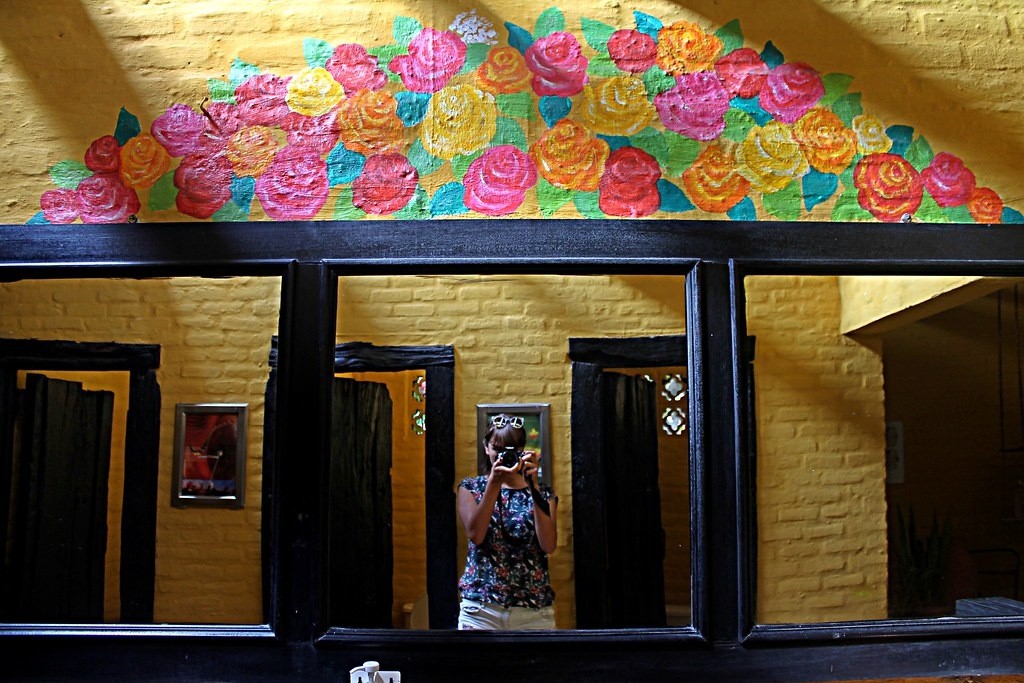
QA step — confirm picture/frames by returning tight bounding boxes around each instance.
[171,403,250,509]
[478,403,554,492]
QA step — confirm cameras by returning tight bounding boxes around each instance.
[498,446,531,475]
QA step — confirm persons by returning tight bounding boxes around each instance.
[457,413,559,629]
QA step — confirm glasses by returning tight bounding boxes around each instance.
[492,414,526,430]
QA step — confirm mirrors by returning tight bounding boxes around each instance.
[1,256,296,644]
[727,259,1024,647]
[309,256,706,650]
